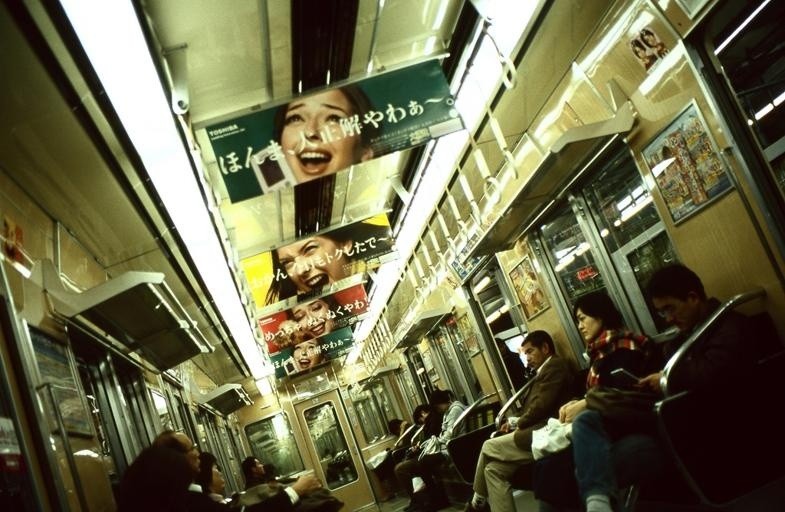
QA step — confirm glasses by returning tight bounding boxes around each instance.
[184,443,199,454]
[657,298,684,319]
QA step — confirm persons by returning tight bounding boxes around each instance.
[269,227,379,294]
[110,427,345,512]
[640,28,669,58]
[631,39,657,70]
[277,86,373,180]
[290,335,325,374]
[384,257,785,510]
[277,296,347,346]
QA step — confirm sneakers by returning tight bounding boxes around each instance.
[411,476,426,493]
[404,496,449,512]
[380,491,396,502]
